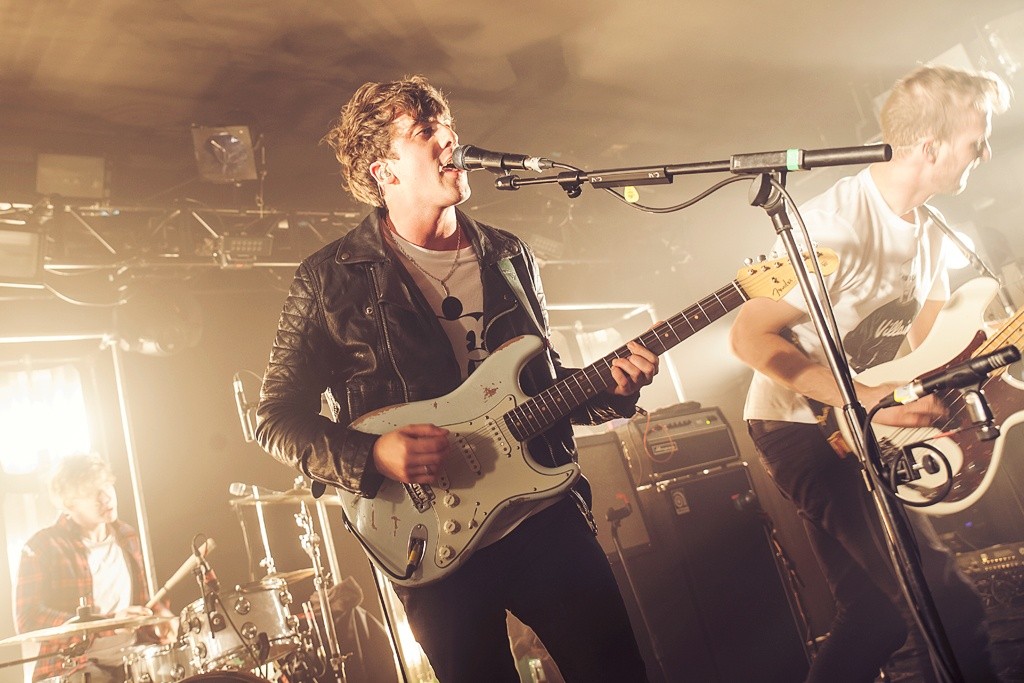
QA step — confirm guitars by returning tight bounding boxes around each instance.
[333,239,843,588]
[870,301,1024,519]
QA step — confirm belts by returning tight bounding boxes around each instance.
[750,420,793,440]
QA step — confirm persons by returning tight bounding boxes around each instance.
[254,74,659,683]
[15,451,179,683]
[729,66,1013,683]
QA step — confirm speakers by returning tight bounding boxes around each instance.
[569,431,816,682]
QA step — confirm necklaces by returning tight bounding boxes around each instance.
[388,222,463,320]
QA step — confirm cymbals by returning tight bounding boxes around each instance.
[0,612,174,650]
[261,563,327,585]
[227,488,341,508]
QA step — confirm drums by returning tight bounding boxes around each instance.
[179,576,300,672]
[120,642,201,683]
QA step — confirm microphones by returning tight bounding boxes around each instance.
[229,483,284,498]
[190,545,220,591]
[604,504,633,520]
[879,344,1022,408]
[451,144,555,173]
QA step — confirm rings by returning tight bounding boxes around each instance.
[426,465,431,474]
